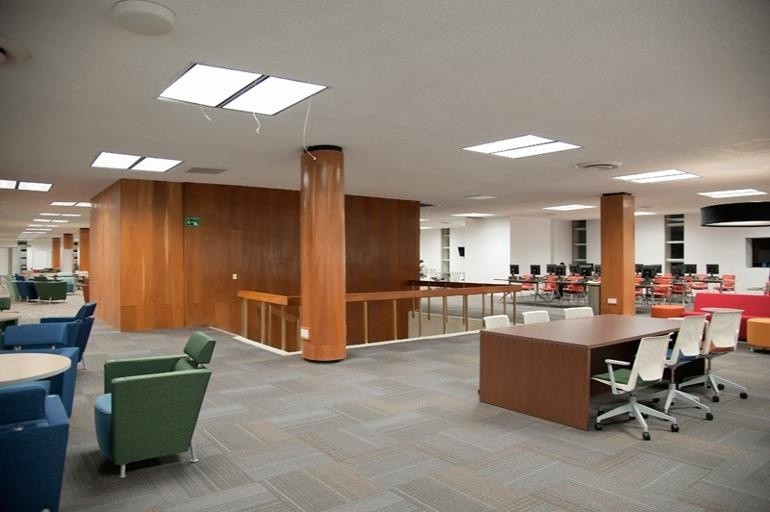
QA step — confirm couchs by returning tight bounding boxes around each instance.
[684,291,770,340]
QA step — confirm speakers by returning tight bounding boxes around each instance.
[458,247,464,257]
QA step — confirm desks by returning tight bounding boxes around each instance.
[0,311,20,326]
[0,353,73,386]
[479,314,712,433]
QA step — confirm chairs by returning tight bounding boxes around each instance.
[493,270,736,310]
[0,297,10,312]
[0,322,80,419]
[483,306,593,330]
[40,302,97,370]
[0,379,70,511]
[93,331,217,479]
[5,272,67,305]
[590,309,750,441]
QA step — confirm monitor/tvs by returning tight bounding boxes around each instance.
[635,264,719,285]
[508,264,601,282]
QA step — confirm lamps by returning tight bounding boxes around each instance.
[700,201,770,227]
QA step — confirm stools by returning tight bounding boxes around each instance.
[651,303,686,318]
[747,317,770,352]
